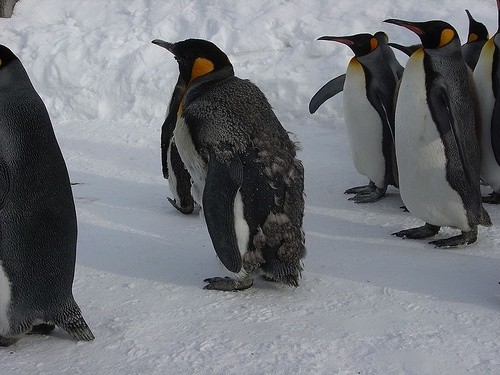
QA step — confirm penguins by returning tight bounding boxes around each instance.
[309,0,500,251]
[147,37,308,292]
[0,45,96,348]
